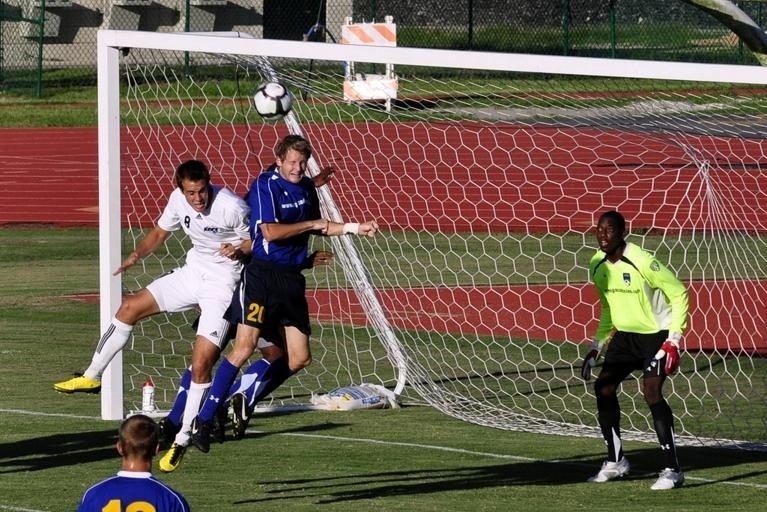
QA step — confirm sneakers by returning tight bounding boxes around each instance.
[52,373,102,395]
[158,393,254,474]
[650,468,684,490]
[587,456,630,483]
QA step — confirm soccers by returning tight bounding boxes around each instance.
[252,81,292,121]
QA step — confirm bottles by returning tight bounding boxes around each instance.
[141,379,156,413]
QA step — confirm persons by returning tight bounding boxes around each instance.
[78,415,191,512]
[582,209,689,493]
[51,161,250,472]
[163,163,341,443]
[192,136,379,453]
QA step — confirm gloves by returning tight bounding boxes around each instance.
[655,332,681,375]
[581,339,604,381]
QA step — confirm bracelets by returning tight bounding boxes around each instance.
[344,223,360,236]
